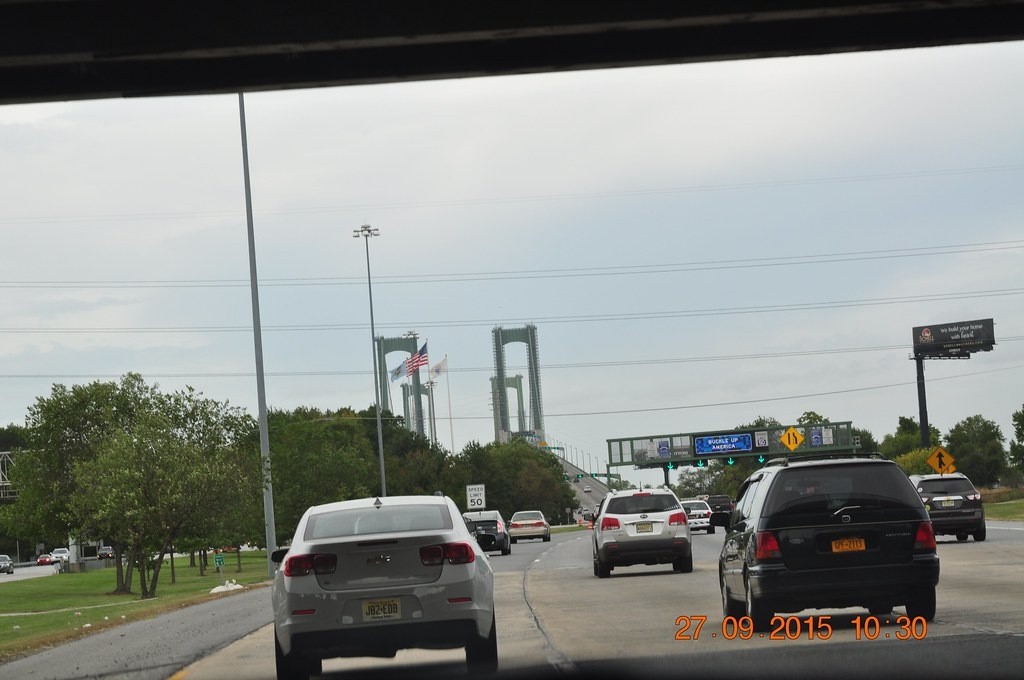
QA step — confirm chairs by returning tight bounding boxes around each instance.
[772,491,801,516]
[654,497,667,509]
[321,516,356,537]
[404,514,443,532]
[612,501,628,515]
[943,483,959,493]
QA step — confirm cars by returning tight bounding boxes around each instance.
[461,510,512,555]
[269,490,499,680]
[37,554,53,566]
[507,510,551,543]
[680,500,715,534]
[96,546,115,560]
[0,555,14,574]
[50,548,70,564]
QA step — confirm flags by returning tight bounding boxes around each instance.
[406,343,428,376]
[389,360,407,382]
[430,357,446,379]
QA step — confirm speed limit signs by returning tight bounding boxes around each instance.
[467,484,487,509]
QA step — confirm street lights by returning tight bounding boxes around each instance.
[352,224,387,497]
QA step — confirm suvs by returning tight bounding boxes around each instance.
[908,472,987,542]
[583,486,694,578]
[706,494,736,527]
[708,448,943,630]
